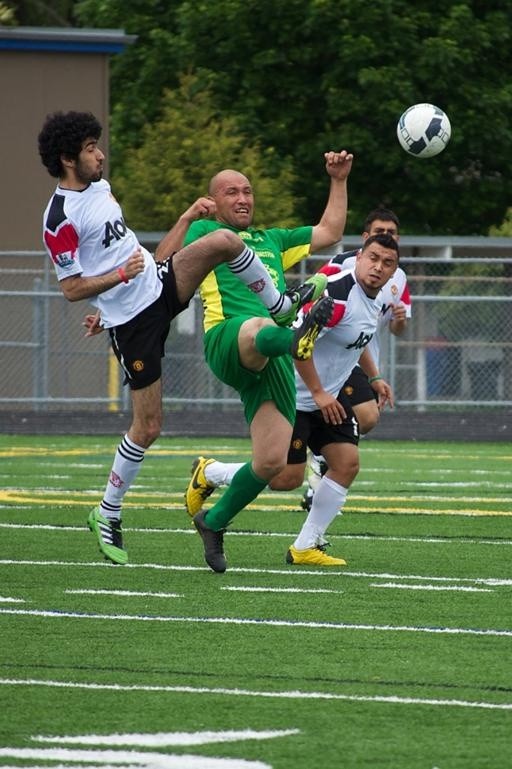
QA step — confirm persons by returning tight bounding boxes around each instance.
[300,204,414,516]
[184,237,400,567]
[154,147,355,573]
[37,110,328,567]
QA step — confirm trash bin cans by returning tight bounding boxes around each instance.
[426,335,512,400]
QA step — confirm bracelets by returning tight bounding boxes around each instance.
[117,266,129,285]
[368,375,383,385]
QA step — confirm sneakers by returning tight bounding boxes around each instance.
[192,508,228,573]
[273,272,328,327]
[184,455,218,518]
[86,505,129,567]
[286,545,346,567]
[301,454,346,518]
[290,293,334,362]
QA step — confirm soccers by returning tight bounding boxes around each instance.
[397,103,451,158]
